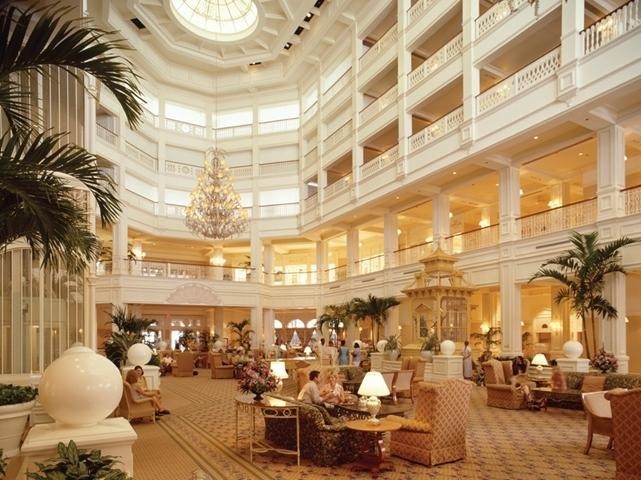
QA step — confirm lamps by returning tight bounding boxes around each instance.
[269,362,289,392]
[183,45,251,240]
[303,346,312,356]
[356,372,391,423]
[532,354,549,376]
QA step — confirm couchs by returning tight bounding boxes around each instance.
[208,350,235,379]
[481,359,536,410]
[387,378,473,467]
[297,366,345,398]
[551,372,641,407]
[262,395,376,466]
[399,356,425,397]
[324,365,367,390]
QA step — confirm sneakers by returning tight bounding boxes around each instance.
[159,409,169,414]
[155,411,160,420]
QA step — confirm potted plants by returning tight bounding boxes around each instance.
[0,382,38,459]
[417,334,438,362]
[382,334,402,361]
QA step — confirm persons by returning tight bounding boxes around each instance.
[126,369,170,417]
[325,341,339,367]
[208,338,255,380]
[351,343,361,368]
[461,341,472,378]
[319,371,344,407]
[549,359,566,389]
[297,371,334,418]
[162,353,173,370]
[317,339,325,356]
[134,366,164,420]
[273,339,292,359]
[513,355,526,375]
[338,340,350,366]
[510,367,537,404]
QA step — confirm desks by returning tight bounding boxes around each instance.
[344,377,364,393]
[345,419,400,479]
[232,393,303,468]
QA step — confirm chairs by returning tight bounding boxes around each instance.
[605,388,640,480]
[509,358,529,386]
[581,390,627,456]
[115,381,156,426]
[379,370,417,405]
[138,376,163,411]
[172,352,193,377]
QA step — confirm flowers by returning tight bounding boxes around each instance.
[588,348,618,371]
[238,359,280,393]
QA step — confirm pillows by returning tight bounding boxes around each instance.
[222,359,231,365]
[307,402,334,425]
[581,376,607,392]
[347,369,361,380]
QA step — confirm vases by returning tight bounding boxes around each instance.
[253,388,265,400]
